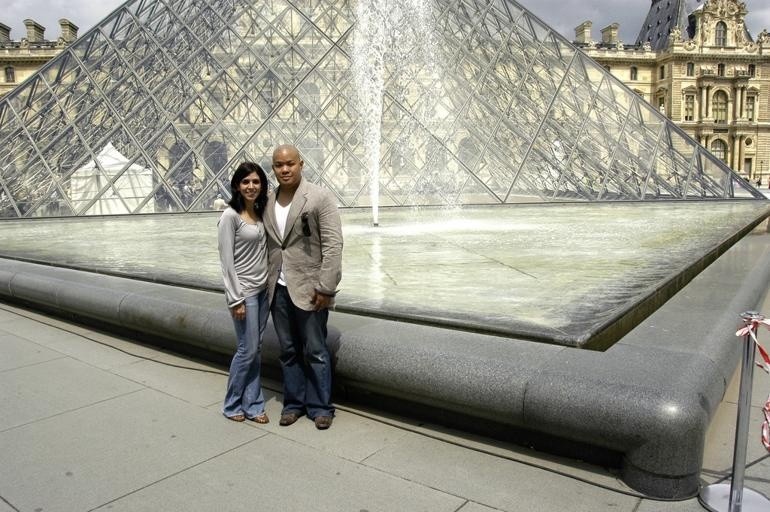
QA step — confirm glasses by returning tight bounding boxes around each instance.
[301,212,312,237]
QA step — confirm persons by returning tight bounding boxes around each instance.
[153,179,233,212]
[218,161,271,425]
[258,144,344,430]
[50,189,59,211]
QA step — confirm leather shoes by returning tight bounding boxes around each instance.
[280,413,298,425]
[252,413,269,423]
[315,415,333,429]
[230,416,245,422]
